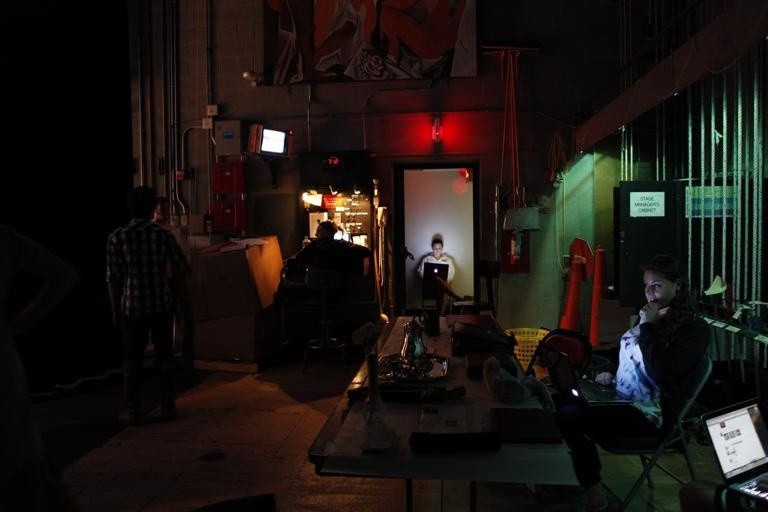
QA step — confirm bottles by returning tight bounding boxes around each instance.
[302,236,311,247]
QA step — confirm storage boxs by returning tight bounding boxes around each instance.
[185,157,372,363]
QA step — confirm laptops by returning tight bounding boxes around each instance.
[560,352,634,407]
[423,263,449,281]
[700,396,768,504]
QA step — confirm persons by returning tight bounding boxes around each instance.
[287,221,371,275]
[544,255,711,512]
[107,186,189,424]
[417,239,470,317]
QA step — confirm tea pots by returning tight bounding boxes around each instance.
[401,315,427,358]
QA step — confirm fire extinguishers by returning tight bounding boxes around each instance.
[510,230,525,265]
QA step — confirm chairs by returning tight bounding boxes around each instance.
[503,328,552,383]
[586,355,714,512]
[422,295,453,315]
[525,328,593,379]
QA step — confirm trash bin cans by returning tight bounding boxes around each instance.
[505,328,551,376]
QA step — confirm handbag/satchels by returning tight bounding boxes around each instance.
[503,207,541,230]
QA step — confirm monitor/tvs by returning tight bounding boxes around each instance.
[624,185,672,226]
[247,124,297,158]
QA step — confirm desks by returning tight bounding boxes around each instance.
[309,313,581,512]
